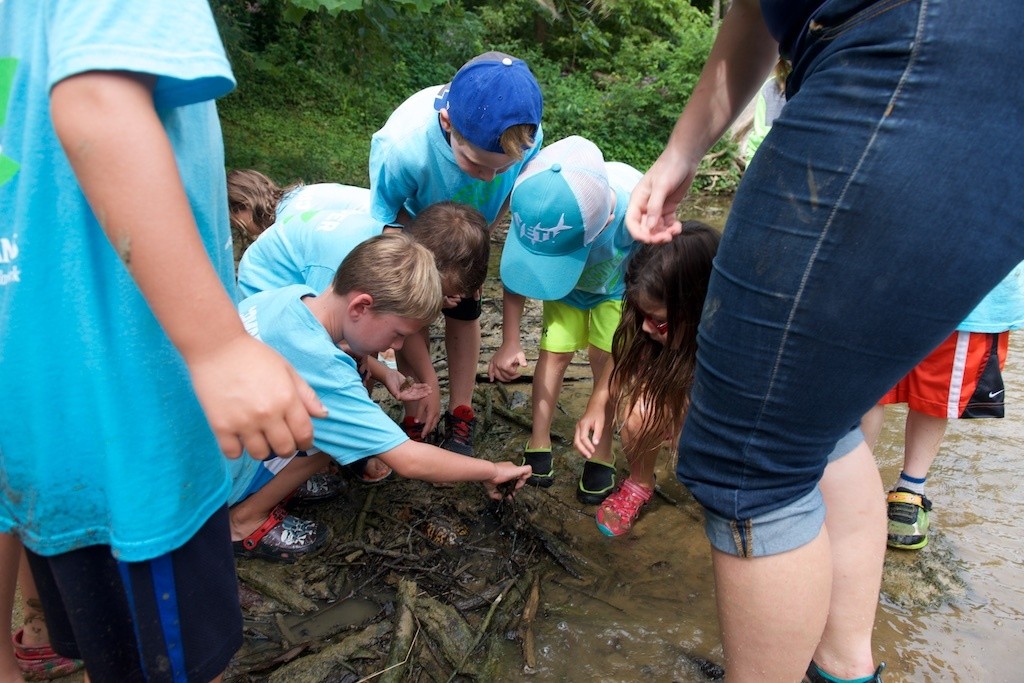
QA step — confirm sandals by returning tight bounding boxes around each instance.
[595,479,653,537]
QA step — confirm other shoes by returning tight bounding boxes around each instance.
[13,628,82,680]
[577,449,618,505]
[521,439,555,488]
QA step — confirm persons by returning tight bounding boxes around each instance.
[626,1,1024,683]
[227,168,370,249]
[237,199,491,483]
[0,0,329,683]
[369,51,544,485]
[860,258,1024,550]
[488,136,647,506]
[227,235,531,562]
[0,531,85,683]
[574,220,722,537]
[731,57,789,162]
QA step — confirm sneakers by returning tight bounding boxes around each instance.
[887,487,934,550]
[439,405,478,459]
[399,416,428,443]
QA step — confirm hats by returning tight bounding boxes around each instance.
[500,134,610,301]
[434,50,544,154]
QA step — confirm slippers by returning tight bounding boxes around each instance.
[287,475,340,502]
[351,456,393,483]
[232,504,327,561]
